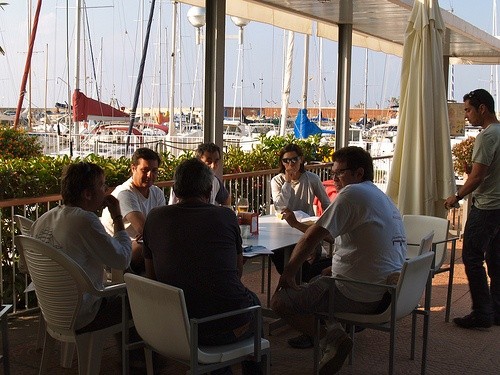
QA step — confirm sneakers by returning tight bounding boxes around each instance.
[288,334,315,348]
[317,327,353,375]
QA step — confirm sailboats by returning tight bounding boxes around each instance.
[0,1,499,167]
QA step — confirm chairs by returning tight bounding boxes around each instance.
[122,270,271,375]
[13,235,154,374]
[396,213,456,325]
[303,252,434,374]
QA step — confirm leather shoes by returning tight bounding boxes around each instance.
[452,312,493,329]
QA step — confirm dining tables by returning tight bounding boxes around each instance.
[124,212,342,349]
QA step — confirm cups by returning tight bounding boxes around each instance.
[240,225,252,248]
[237,198,249,213]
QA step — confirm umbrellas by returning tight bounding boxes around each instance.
[385,1,457,223]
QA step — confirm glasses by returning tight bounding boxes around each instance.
[468,91,481,106]
[330,168,353,177]
[281,158,313,163]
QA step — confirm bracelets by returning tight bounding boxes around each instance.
[455,192,461,202]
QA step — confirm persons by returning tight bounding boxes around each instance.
[168,143,232,214]
[270,146,408,375]
[443,89,500,328]
[98,147,162,360]
[268,145,332,310]
[31,162,165,375]
[143,158,267,375]
